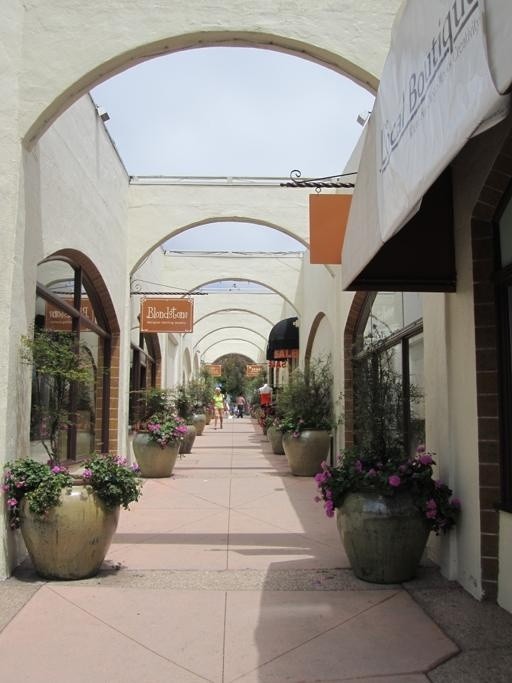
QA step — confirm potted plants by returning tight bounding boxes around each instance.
[269,363,331,478]
[177,379,213,454]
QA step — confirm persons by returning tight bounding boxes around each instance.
[223,394,233,419]
[235,392,247,418]
[210,386,228,431]
[258,383,273,405]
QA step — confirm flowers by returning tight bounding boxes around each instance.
[316,445,458,539]
[2,450,140,532]
[144,414,190,450]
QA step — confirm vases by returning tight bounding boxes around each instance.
[17,480,121,585]
[336,489,433,587]
[133,431,182,480]
